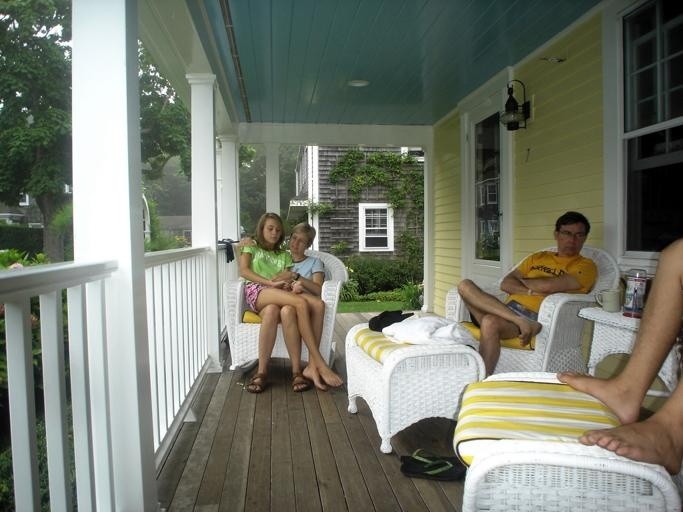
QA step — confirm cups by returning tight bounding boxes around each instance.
[596,290,620,313]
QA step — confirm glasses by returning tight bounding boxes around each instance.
[290,236,309,246]
[557,230,586,240]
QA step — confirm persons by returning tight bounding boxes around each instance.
[244,222,325,394]
[459,212,596,375]
[238,212,342,391]
[557,237,682,475]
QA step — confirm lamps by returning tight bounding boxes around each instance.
[500,79,532,131]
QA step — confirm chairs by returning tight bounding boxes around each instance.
[445,245,620,377]
[224,248,348,373]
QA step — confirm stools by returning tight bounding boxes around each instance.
[342,323,486,454]
[450,370,680,512]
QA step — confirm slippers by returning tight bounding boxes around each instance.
[400,459,466,481]
[401,449,461,465]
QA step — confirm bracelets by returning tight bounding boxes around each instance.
[525,288,533,294]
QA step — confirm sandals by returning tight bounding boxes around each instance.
[292,373,312,392]
[247,373,267,393]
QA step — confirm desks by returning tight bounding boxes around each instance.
[579,306,682,394]
